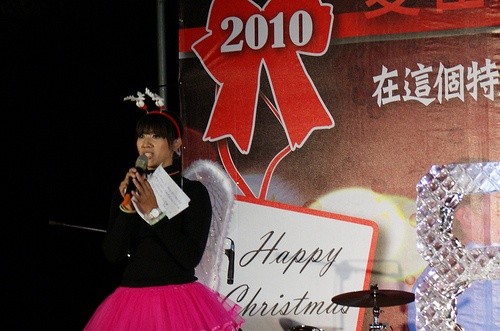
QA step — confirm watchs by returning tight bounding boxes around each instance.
[146,207,162,221]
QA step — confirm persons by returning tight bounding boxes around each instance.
[85,88,245,331]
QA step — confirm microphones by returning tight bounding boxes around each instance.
[123,155,148,205]
